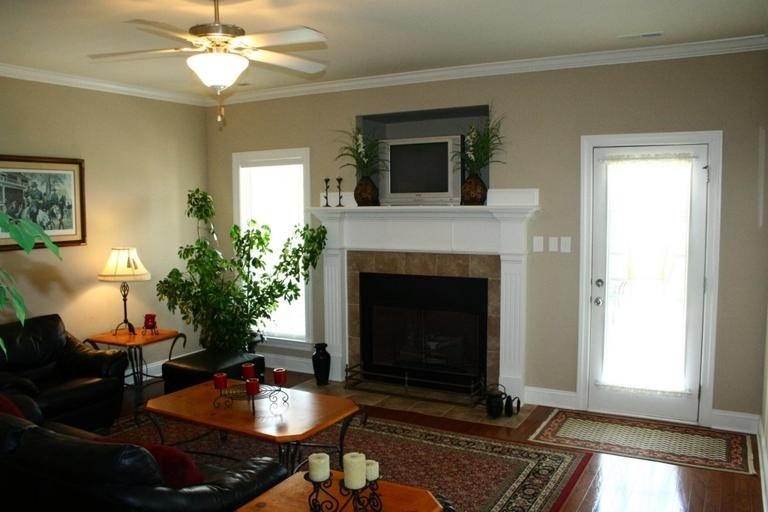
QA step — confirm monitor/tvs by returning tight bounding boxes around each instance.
[377,136,461,207]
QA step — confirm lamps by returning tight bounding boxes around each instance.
[187,52,250,132]
[96,247,151,336]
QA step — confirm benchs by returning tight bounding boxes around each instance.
[162,349,265,394]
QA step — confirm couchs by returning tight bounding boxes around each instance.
[1,393,288,511]
[0,313,129,429]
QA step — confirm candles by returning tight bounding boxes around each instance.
[273,368,285,384]
[213,373,227,389]
[343,452,366,490]
[246,378,259,395]
[145,315,155,328]
[242,363,255,376]
[366,460,378,481]
[308,454,330,482]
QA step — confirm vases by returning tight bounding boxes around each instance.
[461,174,487,206]
[312,343,330,385]
[353,176,378,206]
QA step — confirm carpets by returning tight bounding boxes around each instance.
[528,407,757,475]
[90,404,592,512]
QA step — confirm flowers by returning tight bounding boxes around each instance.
[450,98,508,173]
[334,126,393,178]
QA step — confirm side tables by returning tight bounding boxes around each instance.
[235,470,443,511]
[83,327,187,404]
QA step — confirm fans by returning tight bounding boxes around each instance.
[85,1,329,76]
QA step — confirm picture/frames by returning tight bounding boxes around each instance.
[1,154,86,252]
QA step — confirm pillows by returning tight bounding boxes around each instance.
[39,420,205,488]
[0,394,24,417]
